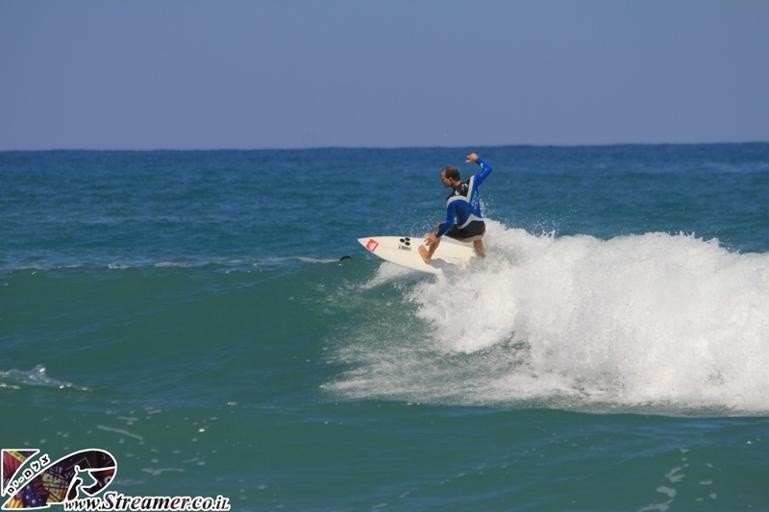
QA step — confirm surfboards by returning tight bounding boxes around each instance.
[358,236,475,275]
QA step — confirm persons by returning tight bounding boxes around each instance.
[418,152,492,264]
